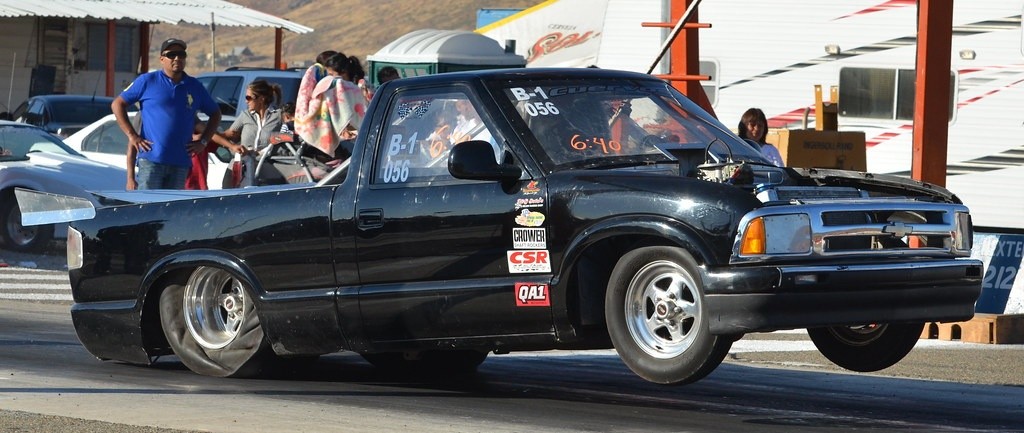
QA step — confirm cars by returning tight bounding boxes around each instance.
[0,61,309,255]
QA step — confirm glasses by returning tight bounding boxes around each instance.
[161,51,186,59]
[246,95,259,100]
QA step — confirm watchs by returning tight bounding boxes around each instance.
[200,139,207,145]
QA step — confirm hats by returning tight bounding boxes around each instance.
[160,38,186,53]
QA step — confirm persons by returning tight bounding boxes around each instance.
[111,38,223,189]
[738,108,784,166]
[127,50,502,191]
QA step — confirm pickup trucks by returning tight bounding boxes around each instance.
[65,65,985,387]
